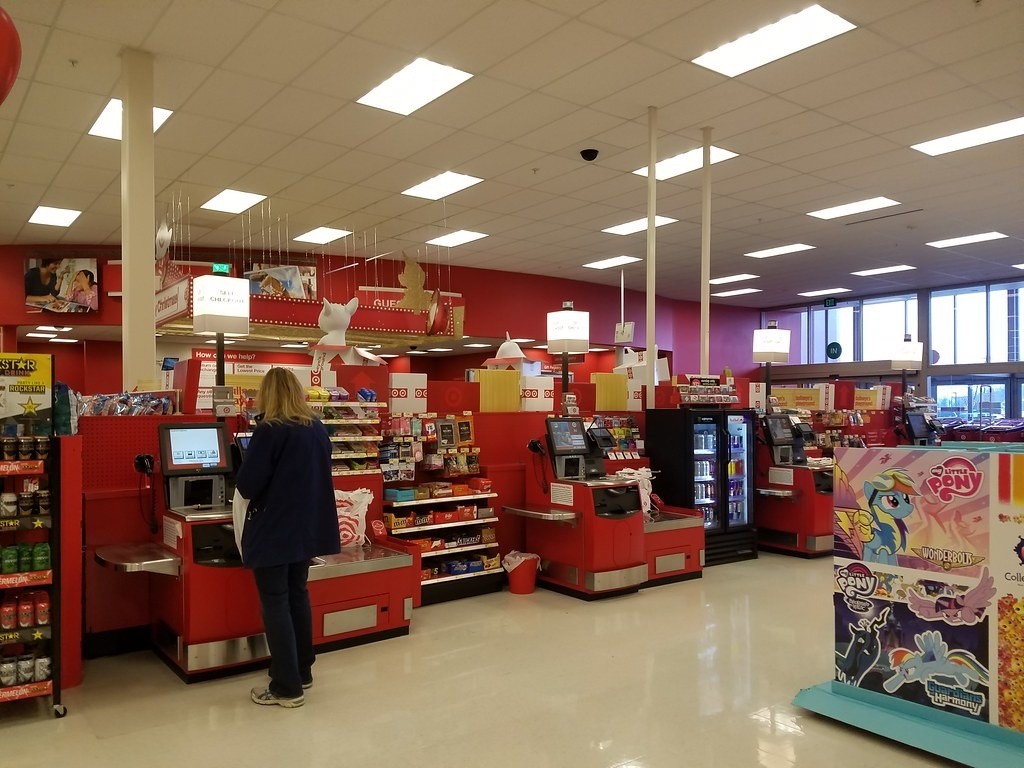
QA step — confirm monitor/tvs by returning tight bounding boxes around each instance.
[764,415,796,446]
[545,418,590,455]
[159,423,233,476]
[907,412,930,438]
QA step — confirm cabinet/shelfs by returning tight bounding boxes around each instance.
[0,355,1024,768]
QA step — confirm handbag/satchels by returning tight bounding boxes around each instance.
[334,487,374,548]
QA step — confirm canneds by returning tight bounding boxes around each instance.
[694,432,741,523]
[0,437,52,688]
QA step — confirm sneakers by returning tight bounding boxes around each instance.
[252,687,305,708]
[302,680,313,689]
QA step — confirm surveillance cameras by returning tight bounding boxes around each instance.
[580,148,599,161]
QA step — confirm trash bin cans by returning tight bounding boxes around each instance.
[505,551,539,595]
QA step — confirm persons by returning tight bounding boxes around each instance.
[229,366,342,709]
[67,269,98,311]
[24,257,70,303]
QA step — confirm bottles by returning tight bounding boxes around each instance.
[830,408,868,426]
[826,430,868,448]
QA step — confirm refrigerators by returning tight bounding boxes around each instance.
[644,407,760,567]
